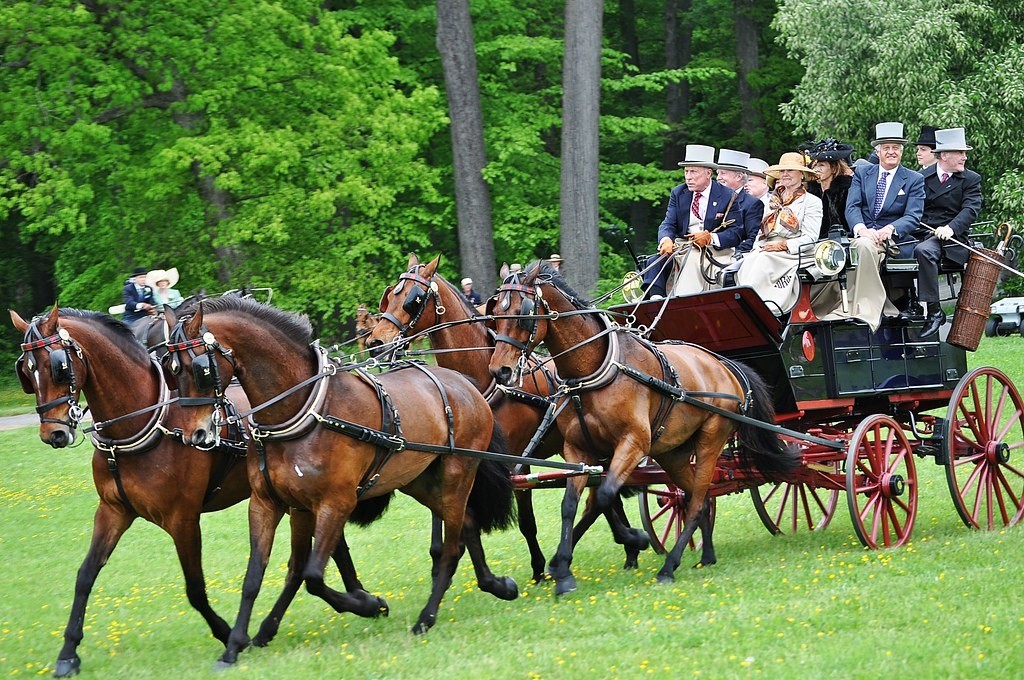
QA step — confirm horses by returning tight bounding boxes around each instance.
[96,390,111,400]
[8,301,390,675]
[489,260,803,596]
[367,251,652,595]
[149,295,520,667]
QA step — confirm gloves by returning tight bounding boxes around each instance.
[764,239,787,252]
[688,230,711,248]
[934,225,954,240]
[659,239,675,257]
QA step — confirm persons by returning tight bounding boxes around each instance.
[145,268,183,311]
[461,278,482,306]
[122,267,158,348]
[547,254,564,269]
[644,122,982,339]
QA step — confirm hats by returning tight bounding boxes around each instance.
[545,254,564,262]
[763,151,817,179]
[461,278,472,286]
[912,126,942,144]
[749,158,769,179]
[931,128,973,152]
[145,267,179,288]
[717,149,752,173]
[678,145,718,169]
[870,122,908,148]
[130,267,148,277]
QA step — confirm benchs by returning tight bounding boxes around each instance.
[636,221,991,324]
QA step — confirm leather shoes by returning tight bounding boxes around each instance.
[918,309,946,340]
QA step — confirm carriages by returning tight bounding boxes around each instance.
[8,232,1023,680]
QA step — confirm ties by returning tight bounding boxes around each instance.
[691,192,704,221]
[873,172,890,219]
[940,173,949,184]
